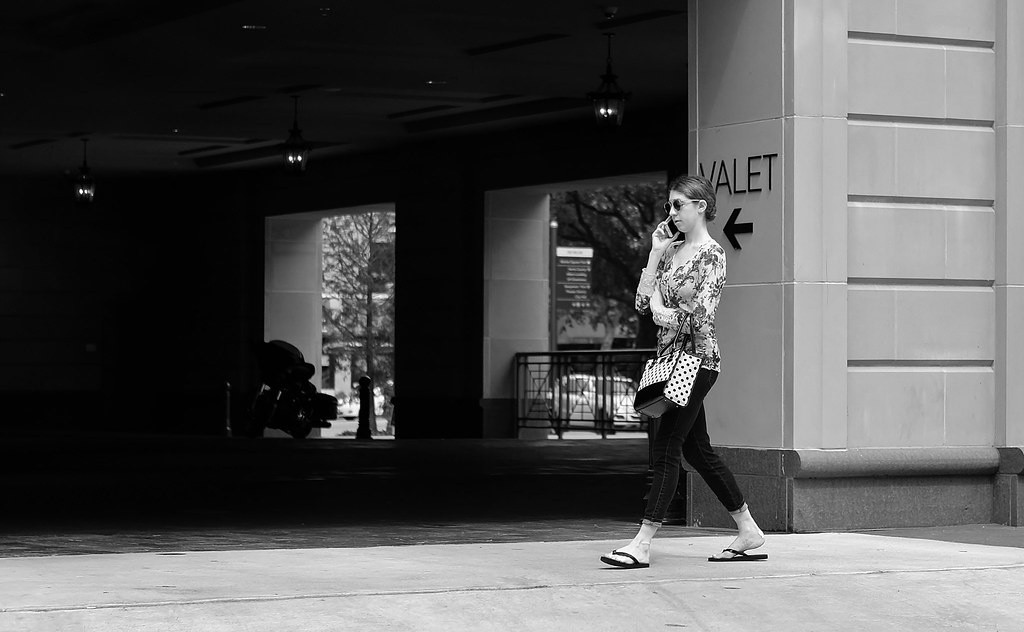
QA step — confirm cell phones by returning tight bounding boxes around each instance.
[665,218,678,238]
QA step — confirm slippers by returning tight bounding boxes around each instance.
[708,548,768,561]
[601,550,649,568]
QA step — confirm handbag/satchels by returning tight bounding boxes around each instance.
[634,313,703,418]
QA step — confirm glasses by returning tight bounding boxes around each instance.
[664,198,700,214]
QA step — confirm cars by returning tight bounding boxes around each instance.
[329,390,385,420]
[545,373,650,433]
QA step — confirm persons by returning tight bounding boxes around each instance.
[601,173,770,568]
